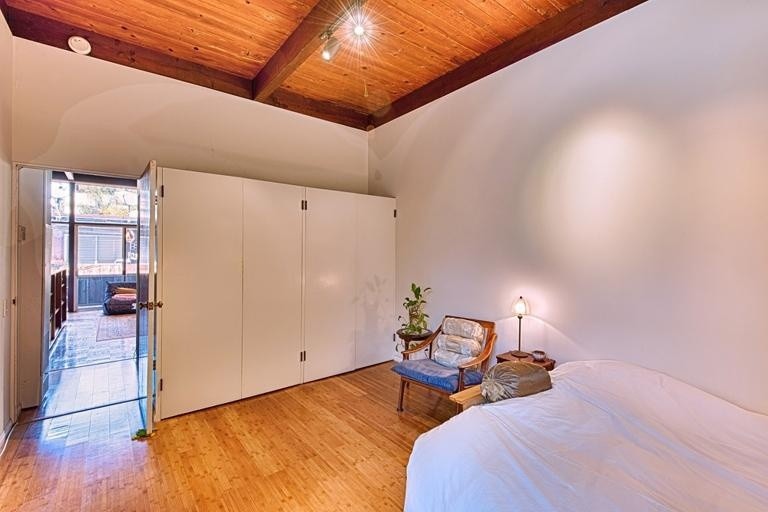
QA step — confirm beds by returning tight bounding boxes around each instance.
[400,355,767,512]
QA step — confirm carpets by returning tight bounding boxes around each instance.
[96,313,148,343]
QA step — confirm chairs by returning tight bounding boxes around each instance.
[102,281,137,315]
[390,315,495,417]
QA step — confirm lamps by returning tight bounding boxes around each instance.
[508,294,530,358]
[317,29,340,61]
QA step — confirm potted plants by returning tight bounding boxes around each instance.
[394,283,428,339]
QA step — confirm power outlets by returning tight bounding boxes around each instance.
[424,345,429,353]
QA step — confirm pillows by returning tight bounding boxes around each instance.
[111,294,136,301]
[432,346,479,370]
[438,333,480,356]
[112,286,137,294]
[441,317,485,343]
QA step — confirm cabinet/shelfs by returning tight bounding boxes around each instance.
[157,166,304,425]
[49,267,68,346]
[303,184,396,383]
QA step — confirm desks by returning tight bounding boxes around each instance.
[395,328,431,388]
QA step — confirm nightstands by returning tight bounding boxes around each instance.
[495,352,554,371]
